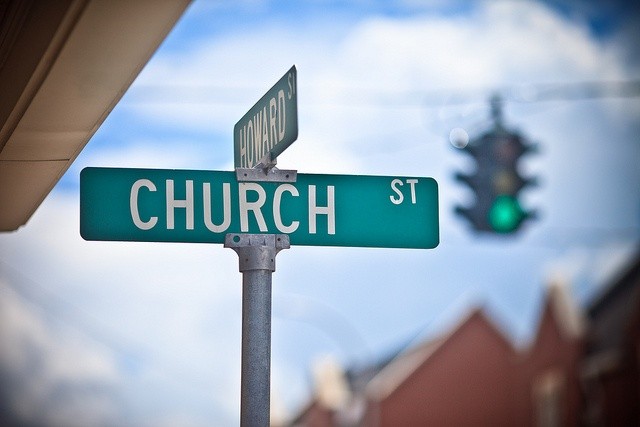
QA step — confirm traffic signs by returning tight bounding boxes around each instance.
[233,64,298,169]
[79,165,438,248]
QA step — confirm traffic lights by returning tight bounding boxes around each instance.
[455,127,539,233]
